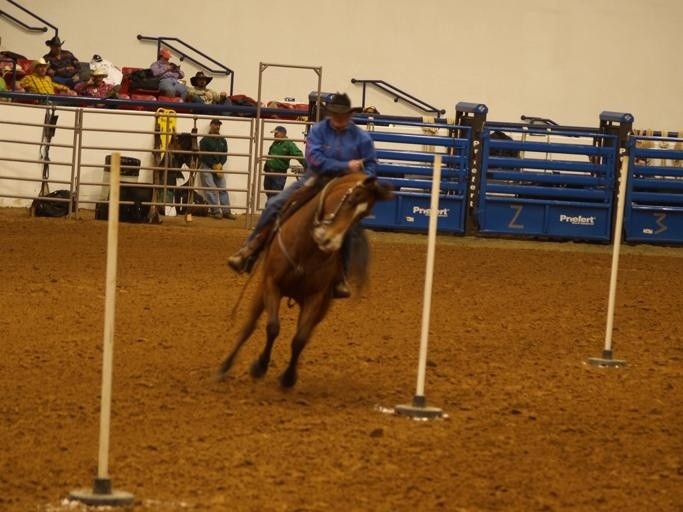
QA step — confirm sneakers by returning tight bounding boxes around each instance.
[229,251,246,270]
[334,282,352,297]
[215,213,236,219]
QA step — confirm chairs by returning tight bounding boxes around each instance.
[0,55,309,120]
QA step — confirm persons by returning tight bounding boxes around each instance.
[0,36,233,115]
[229,92,378,298]
[200,119,236,220]
[263,126,308,201]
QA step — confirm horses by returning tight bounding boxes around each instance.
[212,169,397,393]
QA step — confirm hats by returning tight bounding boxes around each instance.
[271,126,286,133]
[321,94,363,114]
[211,119,222,125]
[91,68,108,77]
[32,58,50,69]
[46,37,64,45]
[191,72,212,85]
[160,48,171,58]
